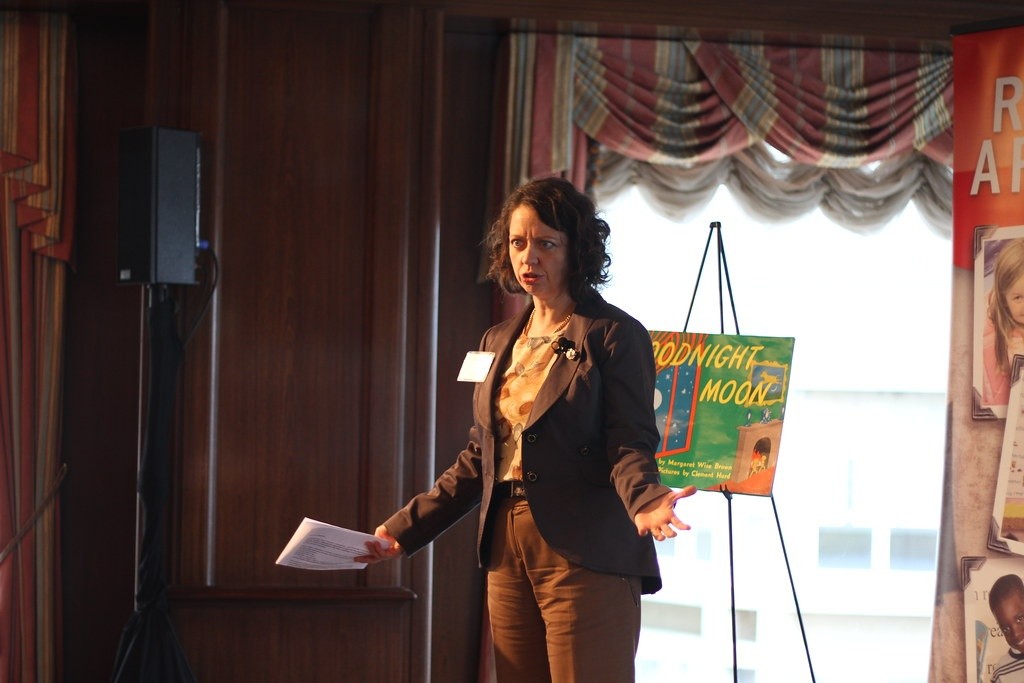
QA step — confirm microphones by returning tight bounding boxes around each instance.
[554,337,568,354]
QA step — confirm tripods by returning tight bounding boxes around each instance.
[111,282,195,682]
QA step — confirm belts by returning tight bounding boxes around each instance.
[496,481,525,498]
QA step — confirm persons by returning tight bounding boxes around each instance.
[347,175,701,682]
[985,572,1024,683]
[982,235,1024,407]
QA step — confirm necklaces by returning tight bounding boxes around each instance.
[523,304,575,337]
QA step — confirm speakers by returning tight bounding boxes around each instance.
[118,124,202,285]
